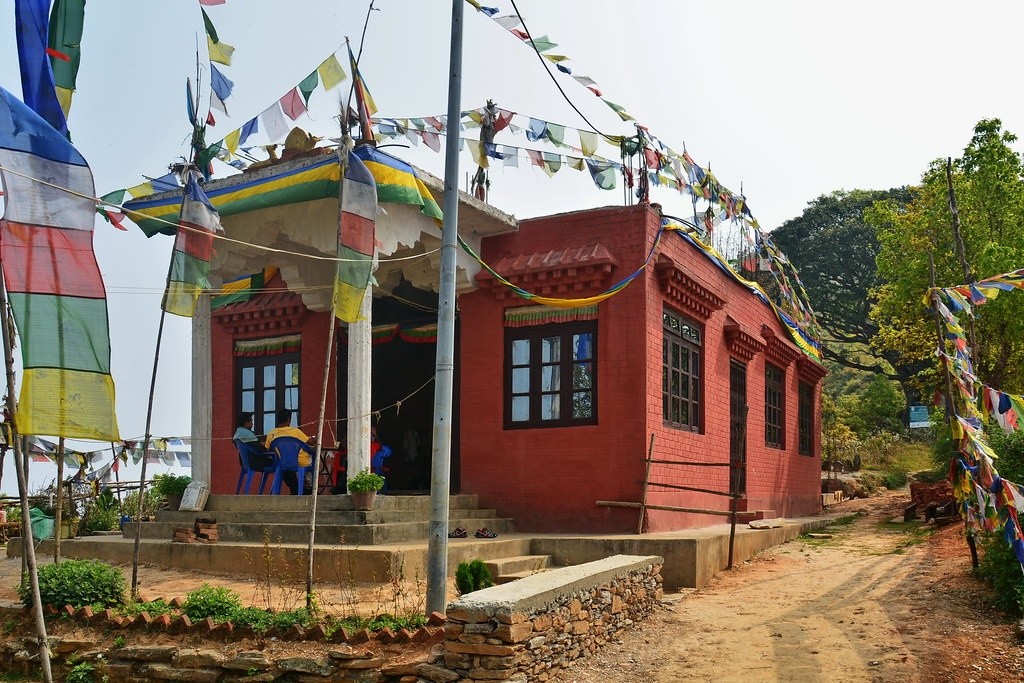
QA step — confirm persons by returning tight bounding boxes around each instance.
[233,412,310,495]
[331,426,381,495]
[264,409,315,467]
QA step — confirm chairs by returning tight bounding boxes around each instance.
[233,439,272,495]
[333,445,390,487]
[267,437,314,495]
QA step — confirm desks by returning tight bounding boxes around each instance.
[307,445,338,496]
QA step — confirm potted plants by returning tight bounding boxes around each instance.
[348,467,384,512]
[158,474,193,512]
